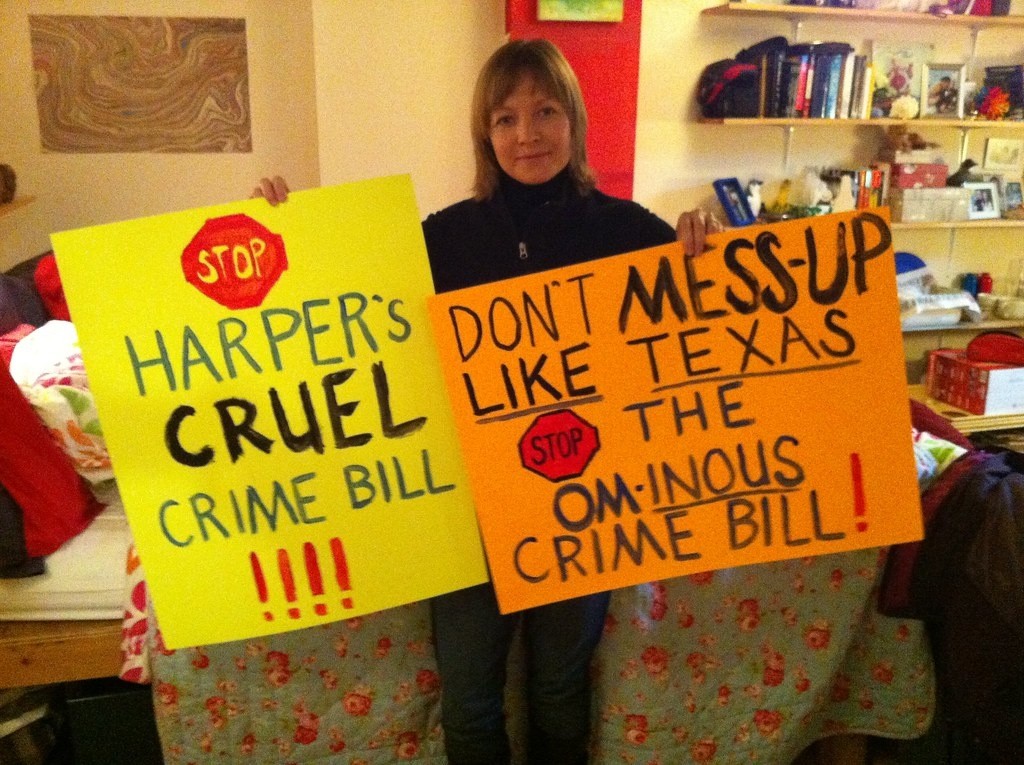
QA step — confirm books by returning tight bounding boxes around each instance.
[822,162,891,208]
[901,309,962,329]
[909,383,1024,432]
[757,50,876,120]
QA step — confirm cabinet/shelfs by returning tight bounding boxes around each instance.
[700,3,1024,435]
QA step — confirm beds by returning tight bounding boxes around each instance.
[0,194,135,689]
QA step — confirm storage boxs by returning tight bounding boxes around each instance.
[880,148,946,189]
[889,188,969,223]
[927,349,1023,416]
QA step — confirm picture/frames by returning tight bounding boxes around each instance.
[961,182,1001,221]
[983,137,1022,172]
[1002,178,1023,213]
[872,39,926,100]
[983,173,1008,214]
[919,59,967,119]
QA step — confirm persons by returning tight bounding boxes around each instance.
[250,37,726,765]
[931,77,957,113]
[975,191,993,211]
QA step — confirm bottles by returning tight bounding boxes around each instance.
[978,271,993,294]
[964,273,978,298]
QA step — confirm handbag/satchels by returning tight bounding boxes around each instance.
[697,58,760,118]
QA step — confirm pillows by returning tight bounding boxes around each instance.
[1,322,106,559]
[10,320,121,504]
[35,252,71,322]
